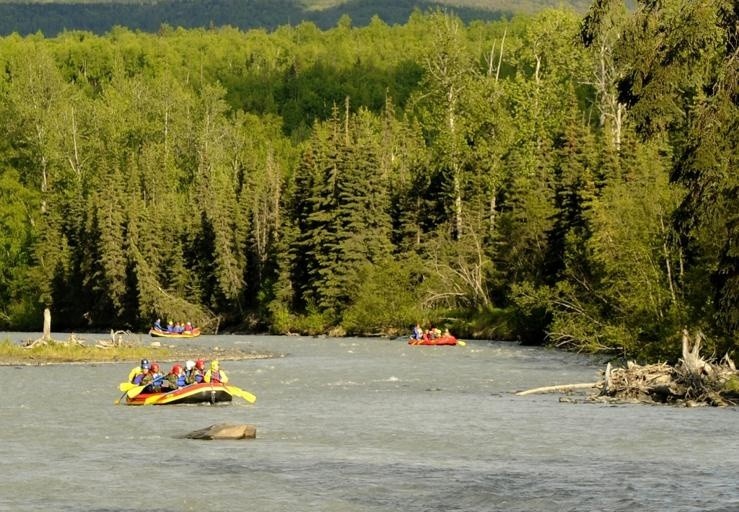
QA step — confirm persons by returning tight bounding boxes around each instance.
[142,363,169,384]
[194,360,208,384]
[153,364,186,389]
[153,318,194,336]
[127,357,150,383]
[176,360,196,387]
[203,360,229,385]
[133,363,151,386]
[413,323,450,341]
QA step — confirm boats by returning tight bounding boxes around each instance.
[125,367,234,405]
[408,333,456,346]
[148,327,203,342]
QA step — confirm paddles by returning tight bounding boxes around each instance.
[115,372,170,404]
[211,377,257,404]
[457,340,466,345]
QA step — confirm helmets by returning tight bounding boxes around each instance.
[168,321,193,326]
[211,360,220,368]
[142,359,148,364]
[142,364,149,369]
[173,365,183,375]
[196,361,204,367]
[186,361,196,370]
[151,364,159,371]
